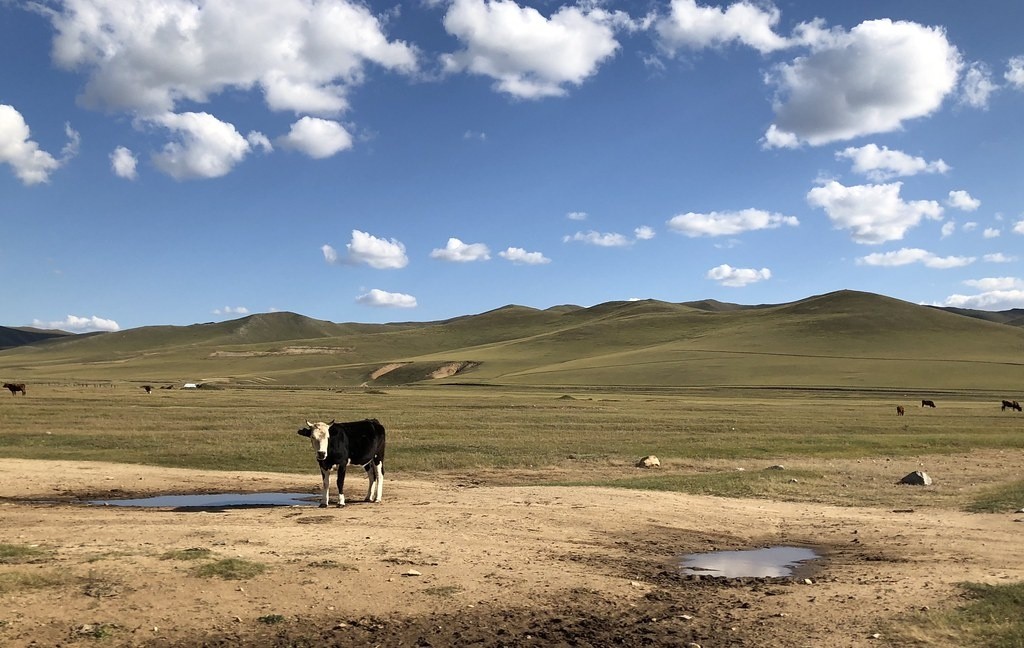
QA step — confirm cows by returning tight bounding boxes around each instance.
[897,405,905,416]
[1001,399,1023,412]
[297,418,386,509]
[3,383,26,396]
[922,399,936,408]
